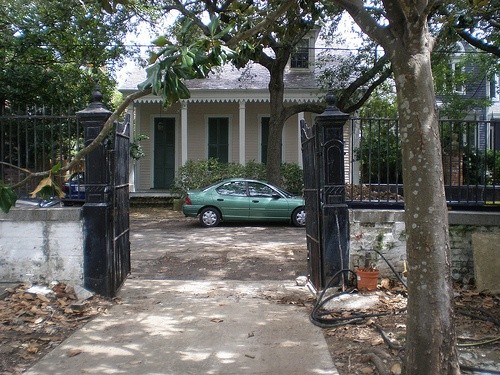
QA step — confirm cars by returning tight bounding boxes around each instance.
[61,171,90,206]
[181,178,307,227]
